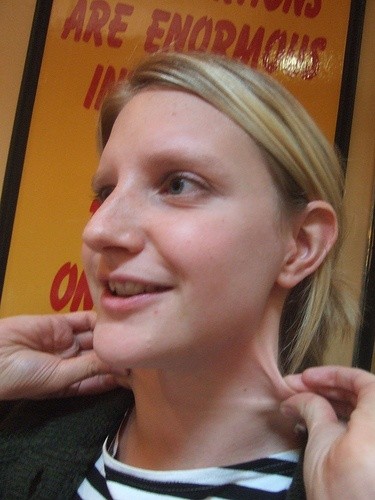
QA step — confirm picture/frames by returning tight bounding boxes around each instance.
[1,0,375,367]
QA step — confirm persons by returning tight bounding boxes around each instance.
[1,48,375,500]
